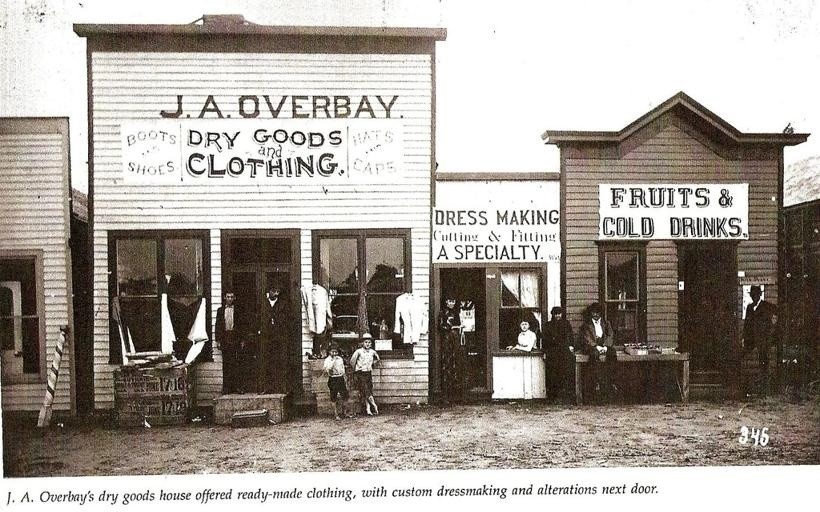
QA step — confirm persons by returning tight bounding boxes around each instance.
[256,280,293,395]
[505,318,538,352]
[349,332,381,415]
[578,301,621,393]
[541,306,577,407]
[323,342,356,422]
[437,291,470,403]
[729,284,785,397]
[214,288,249,395]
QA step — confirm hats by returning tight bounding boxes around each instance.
[748,285,764,293]
[362,332,373,341]
[327,343,339,352]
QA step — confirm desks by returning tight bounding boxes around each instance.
[573,349,691,405]
